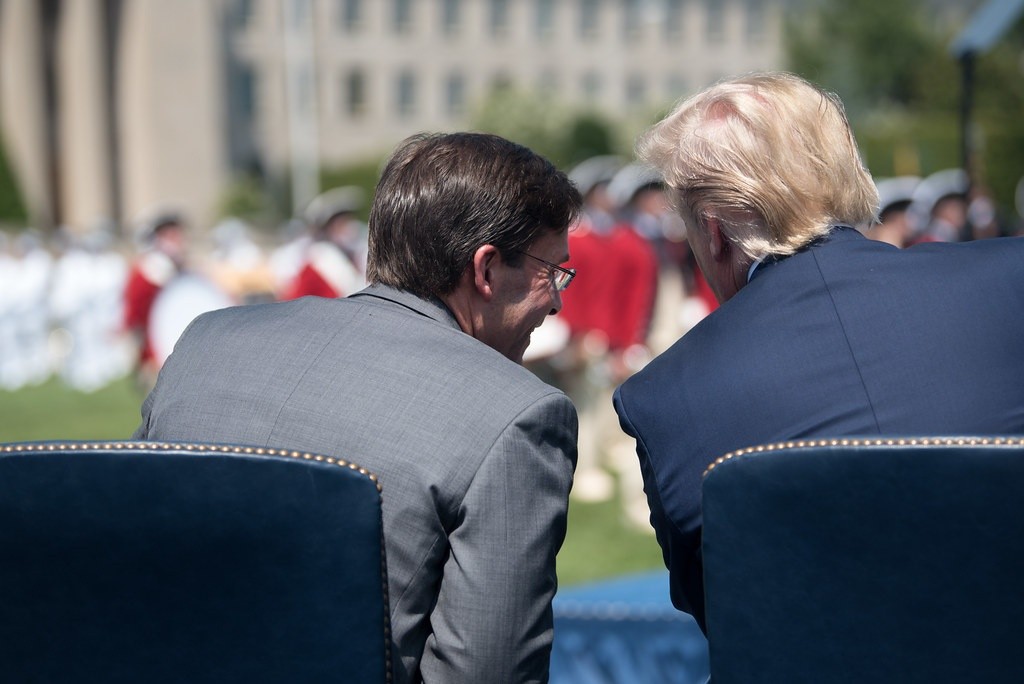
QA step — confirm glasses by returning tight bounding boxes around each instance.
[512,248,576,292]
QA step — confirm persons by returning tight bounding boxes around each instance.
[0,156,976,532]
[128,133,585,684]
[612,68,1024,642]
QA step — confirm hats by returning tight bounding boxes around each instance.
[566,151,624,202]
[136,208,191,242]
[870,174,917,228]
[906,168,972,229]
[304,184,364,231]
[608,164,664,213]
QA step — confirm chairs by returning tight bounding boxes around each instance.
[0,440,391,684]
[702,431,1024,684]
